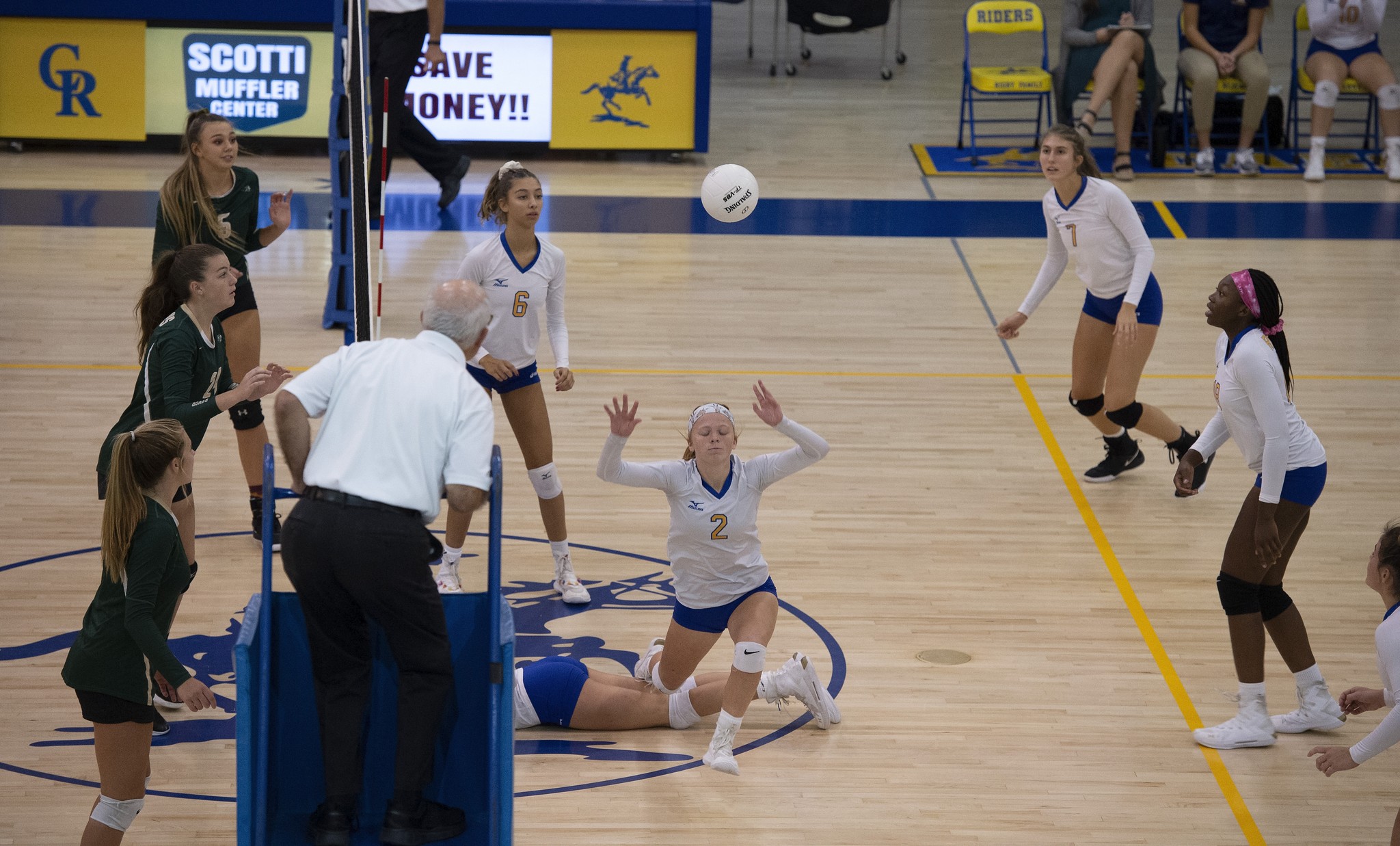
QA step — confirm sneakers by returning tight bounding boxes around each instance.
[1270,679,1346,734]
[1164,430,1216,498]
[553,568,591,603]
[702,722,740,776]
[1083,436,1146,483]
[435,571,463,594]
[634,637,665,693]
[1192,691,1277,749]
[251,512,283,552]
[774,651,840,729]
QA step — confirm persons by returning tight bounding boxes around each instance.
[1304,0,1400,181]
[1172,269,1347,751]
[274,285,497,846]
[416,161,593,605]
[95,244,294,737]
[350,0,471,222]
[596,379,831,773]
[1173,0,1272,172]
[507,653,843,731]
[995,124,1216,498]
[60,418,217,846]
[1056,0,1154,181]
[151,111,293,556]
[1307,520,1400,846]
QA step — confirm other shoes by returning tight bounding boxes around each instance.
[1231,148,1259,175]
[437,153,471,207]
[1381,145,1400,181]
[1192,147,1216,176]
[150,687,185,709]
[1303,144,1326,181]
[149,705,171,736]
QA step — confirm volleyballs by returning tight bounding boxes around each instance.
[701,163,760,223]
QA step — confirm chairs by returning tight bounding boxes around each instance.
[1169,6,1270,166]
[957,0,1055,166]
[1074,76,1153,164]
[1284,1,1383,165]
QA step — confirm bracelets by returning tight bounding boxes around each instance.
[1183,450,1203,469]
[427,40,440,47]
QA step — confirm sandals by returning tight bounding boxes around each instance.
[1077,109,1098,135]
[1111,151,1134,179]
